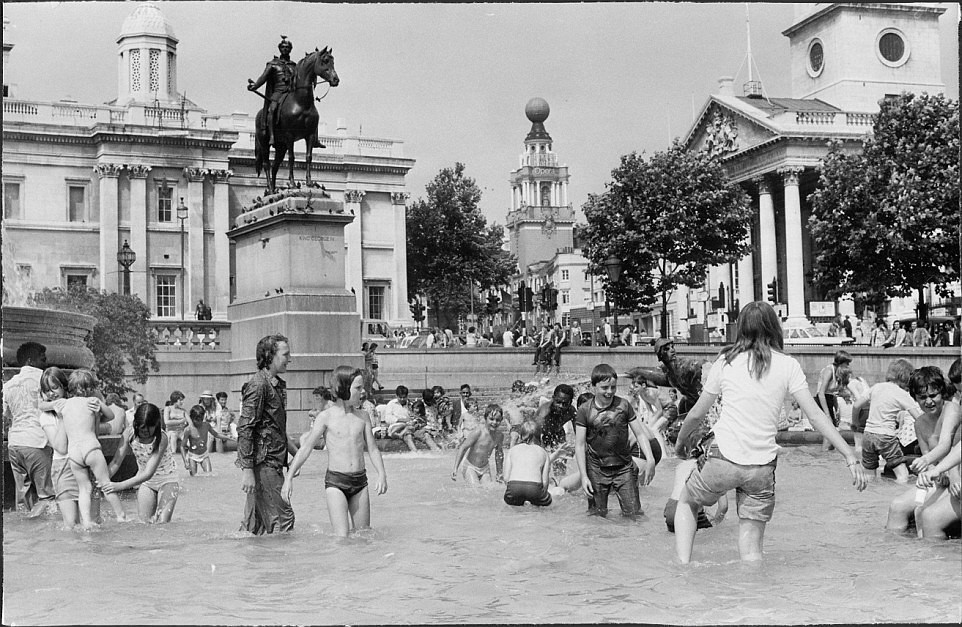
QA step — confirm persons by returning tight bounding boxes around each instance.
[247,34,327,148]
[3,300,962,564]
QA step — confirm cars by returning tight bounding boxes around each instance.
[782,326,855,347]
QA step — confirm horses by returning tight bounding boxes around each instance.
[253,46,339,194]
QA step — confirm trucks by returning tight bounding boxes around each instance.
[361,320,397,349]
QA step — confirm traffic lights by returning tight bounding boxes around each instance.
[410,305,419,323]
[527,287,534,311]
[541,287,550,311]
[550,289,559,309]
[486,296,492,314]
[767,281,777,304]
[518,288,525,312]
[418,304,425,321]
[492,297,500,313]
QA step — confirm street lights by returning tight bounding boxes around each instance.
[177,198,189,320]
[604,254,626,346]
[116,239,136,298]
